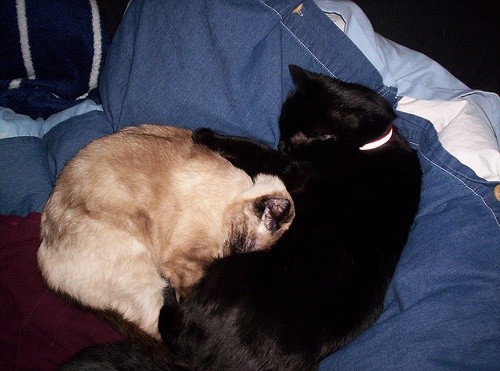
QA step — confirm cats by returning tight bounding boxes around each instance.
[46,62,425,370]
[36,121,297,343]
